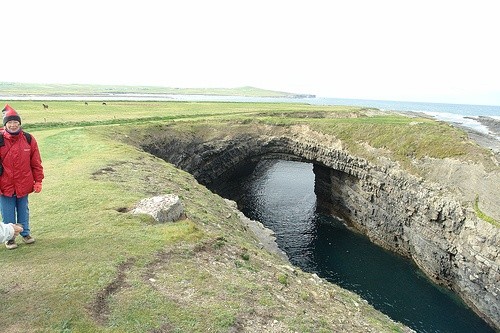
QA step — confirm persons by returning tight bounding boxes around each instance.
[0,220,24,244]
[0,104,45,250]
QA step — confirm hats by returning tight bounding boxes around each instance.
[2,104,21,125]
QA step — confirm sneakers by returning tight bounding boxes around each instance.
[4,238,18,249]
[21,234,34,243]
